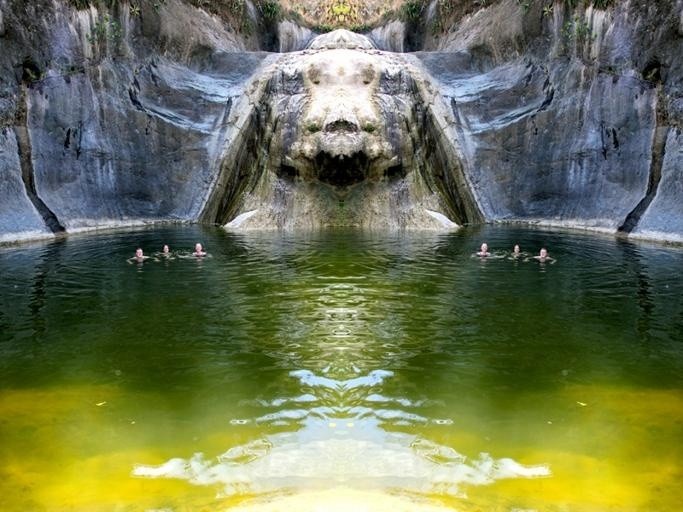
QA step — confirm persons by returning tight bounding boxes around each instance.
[534,247,556,264]
[192,242,207,255]
[477,242,490,256]
[125,247,159,265]
[162,244,169,253]
[512,243,521,253]
